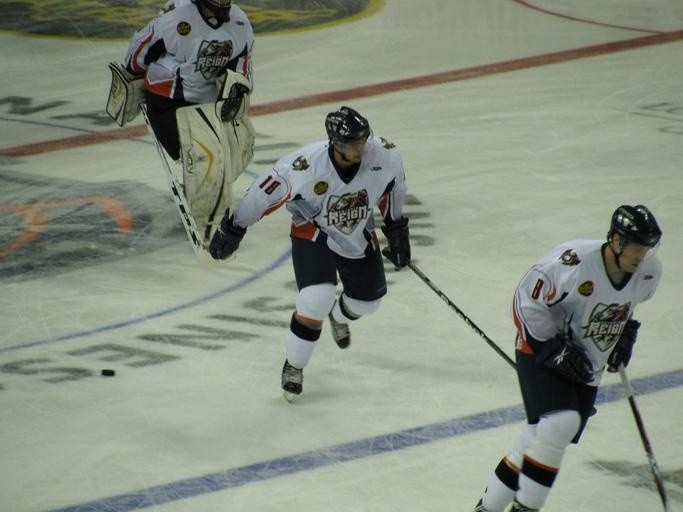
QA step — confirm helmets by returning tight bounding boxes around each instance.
[190,0,231,23]
[607,204,663,247]
[324,106,371,146]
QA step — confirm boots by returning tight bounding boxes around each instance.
[328,299,351,349]
[281,358,304,403]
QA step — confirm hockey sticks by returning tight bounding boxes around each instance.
[404,257,597,417]
[133,101,239,266]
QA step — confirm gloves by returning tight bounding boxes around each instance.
[534,333,595,385]
[382,216,411,268]
[209,208,248,260]
[607,319,642,373]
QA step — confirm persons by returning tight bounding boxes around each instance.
[124,0,256,161]
[471,203,665,512]
[208,103,412,397]
